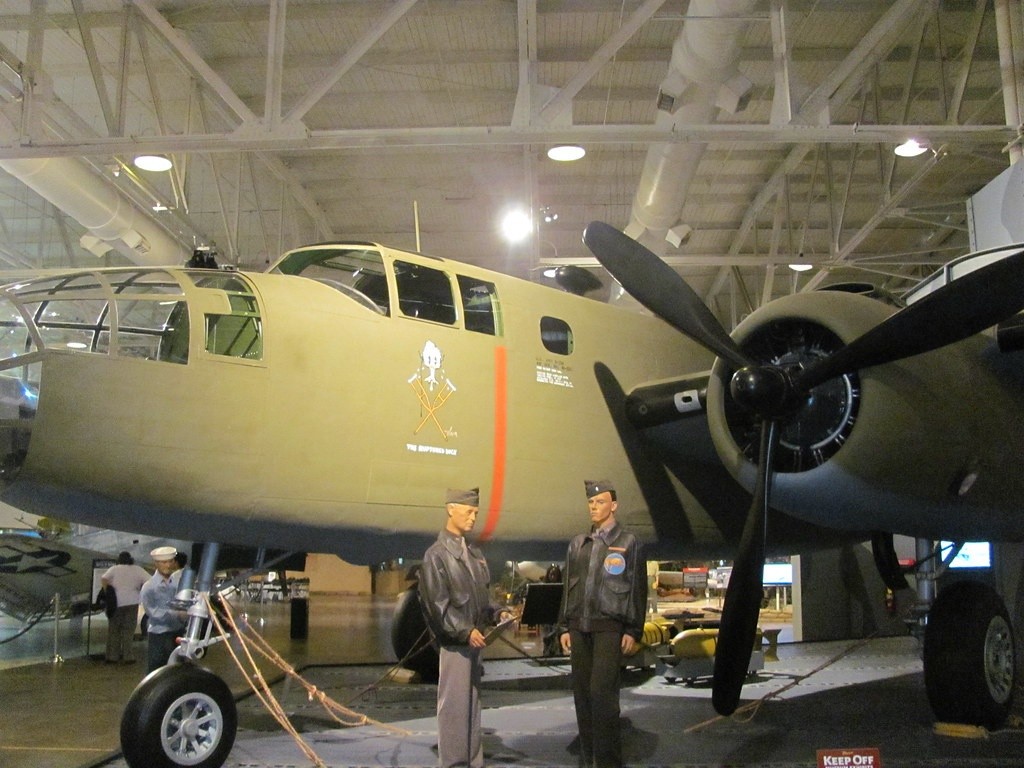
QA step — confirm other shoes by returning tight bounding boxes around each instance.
[106,660,118,664]
[125,660,135,664]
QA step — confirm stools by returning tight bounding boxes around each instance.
[517,604,539,637]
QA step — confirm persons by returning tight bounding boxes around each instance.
[421,487,517,768]
[140,546,193,671]
[559,479,648,768]
[101,551,152,663]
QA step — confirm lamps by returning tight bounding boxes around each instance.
[548,144,586,162]
[134,154,173,172]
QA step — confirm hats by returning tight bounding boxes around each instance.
[445,487,479,506]
[584,479,615,498]
[150,546,177,561]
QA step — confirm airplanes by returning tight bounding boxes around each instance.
[0,214,1024,768]
